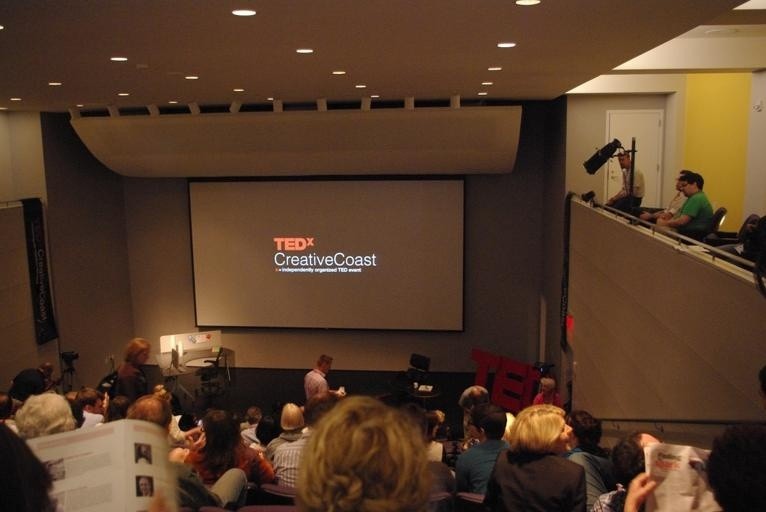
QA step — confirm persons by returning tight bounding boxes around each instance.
[634,169,694,222]
[602,152,646,215]
[656,173,713,233]
[2,338,766,512]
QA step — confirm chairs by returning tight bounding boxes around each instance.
[391,353,430,402]
[670,206,728,246]
[194,347,223,392]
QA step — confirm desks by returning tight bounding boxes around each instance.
[409,389,441,410]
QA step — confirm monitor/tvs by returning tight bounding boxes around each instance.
[165,349,179,372]
[61,350,79,373]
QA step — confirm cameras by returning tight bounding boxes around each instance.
[533,361,556,377]
